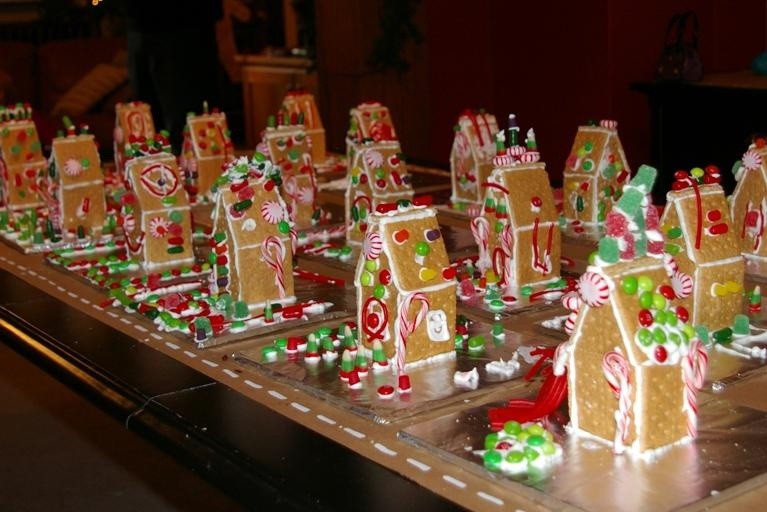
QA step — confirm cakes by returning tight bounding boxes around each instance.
[449,109,501,214]
[255,110,332,230]
[487,164,712,465]
[354,194,459,371]
[281,89,326,163]
[29,114,109,236]
[180,101,237,205]
[210,150,297,310]
[0,102,49,216]
[117,130,195,271]
[561,118,633,240]
[470,112,562,288]
[345,100,399,179]
[113,100,155,177]
[343,142,416,244]
[726,138,767,269]
[660,162,749,345]
